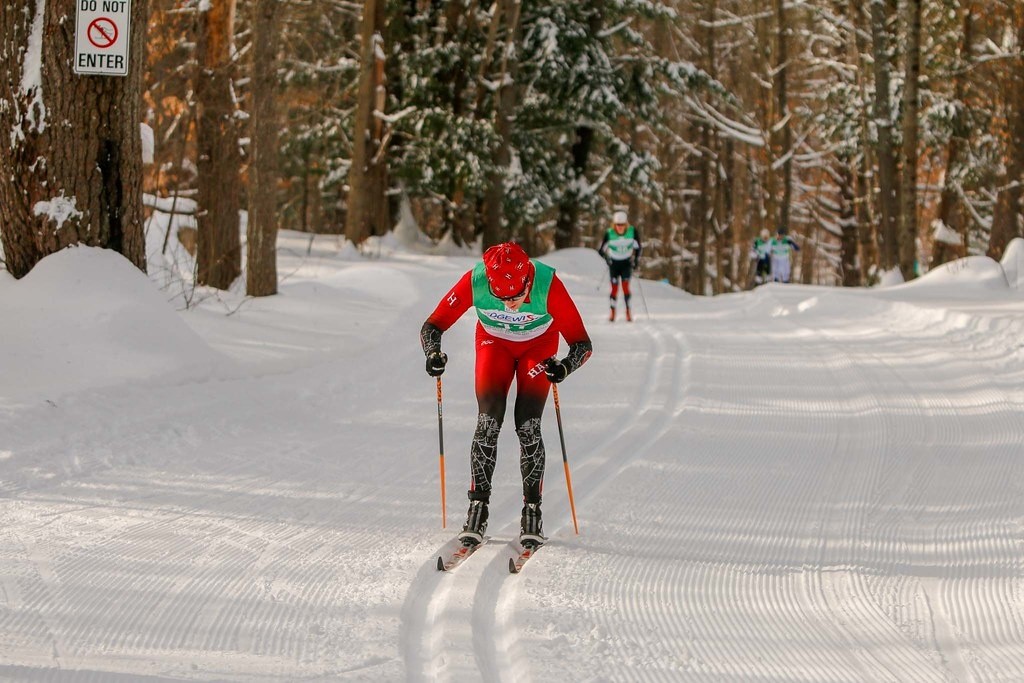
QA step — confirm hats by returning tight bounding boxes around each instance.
[483,242,529,298]
[614,212,627,224]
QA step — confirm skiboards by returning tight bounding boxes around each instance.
[436,534,548,575]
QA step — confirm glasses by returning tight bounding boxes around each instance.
[488,277,529,301]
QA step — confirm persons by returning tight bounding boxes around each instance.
[598,211,643,322]
[750,226,801,286]
[419,241,593,550]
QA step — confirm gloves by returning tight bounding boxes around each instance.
[426,352,448,377]
[543,359,572,383]
[599,250,605,257]
[634,261,638,270]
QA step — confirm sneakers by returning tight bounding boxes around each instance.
[459,503,488,543]
[519,507,544,547]
